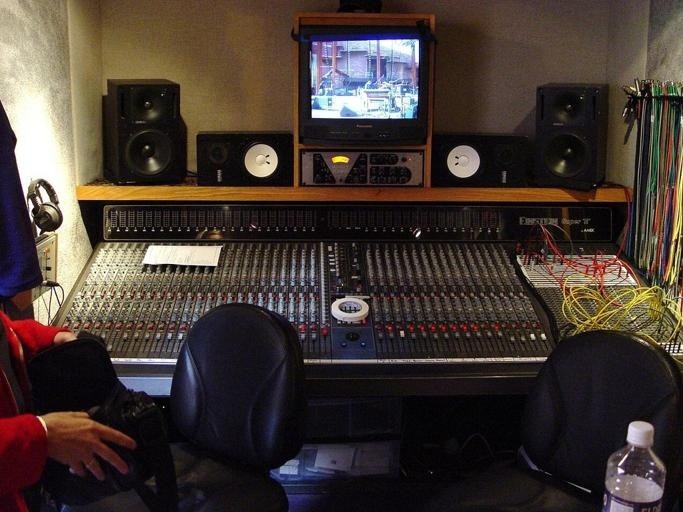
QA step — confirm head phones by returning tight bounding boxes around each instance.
[27,179,63,231]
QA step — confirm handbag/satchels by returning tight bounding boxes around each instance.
[514,327,683,512]
[21,332,173,506]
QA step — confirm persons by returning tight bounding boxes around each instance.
[0,304,140,509]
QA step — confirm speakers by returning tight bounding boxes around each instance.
[196,130,294,186]
[535,82,609,191]
[431,132,533,188]
[107,79,181,187]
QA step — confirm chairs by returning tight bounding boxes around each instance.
[409,330,680,511]
[67,304,306,511]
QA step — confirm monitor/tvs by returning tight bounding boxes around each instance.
[298,24,430,147]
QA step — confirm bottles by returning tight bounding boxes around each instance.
[601,420,667,512]
